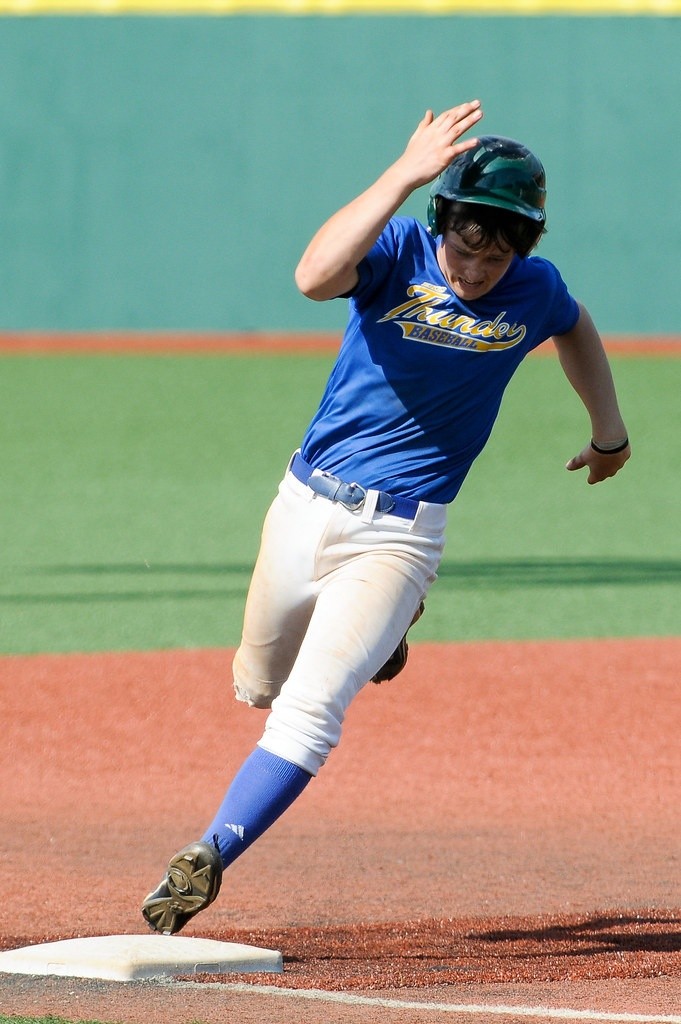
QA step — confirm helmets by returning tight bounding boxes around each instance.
[427,135,546,238]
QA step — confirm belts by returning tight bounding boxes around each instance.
[290,452,419,520]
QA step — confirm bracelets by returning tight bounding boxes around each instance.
[591,438,629,453]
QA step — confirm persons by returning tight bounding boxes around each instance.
[142,100,631,935]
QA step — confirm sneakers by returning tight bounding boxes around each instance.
[142,833,223,936]
[370,600,425,684]
